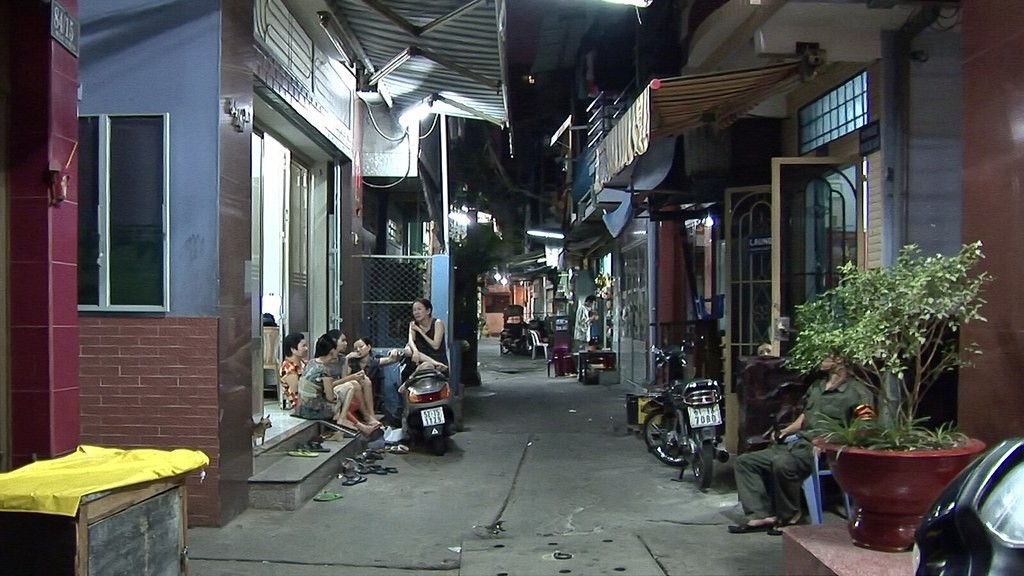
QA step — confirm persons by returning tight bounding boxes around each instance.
[296,334,359,431]
[347,338,398,421]
[573,296,599,350]
[279,332,308,410]
[326,329,383,426]
[728,354,877,535]
[398,299,449,395]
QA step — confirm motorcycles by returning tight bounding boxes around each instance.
[639,335,730,491]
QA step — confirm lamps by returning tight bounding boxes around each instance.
[526,227,566,239]
[396,93,442,131]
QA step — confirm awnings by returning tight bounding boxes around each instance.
[328,0,513,158]
[594,58,801,196]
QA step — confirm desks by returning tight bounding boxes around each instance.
[578,350,616,382]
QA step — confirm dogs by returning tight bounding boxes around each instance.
[250,413,273,448]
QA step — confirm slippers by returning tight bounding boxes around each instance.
[728,524,767,533]
[297,442,330,452]
[288,448,319,457]
[313,491,343,501]
[768,511,803,535]
[341,475,366,486]
[341,444,409,478]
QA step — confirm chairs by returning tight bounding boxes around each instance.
[773,431,853,525]
[530,331,548,360]
[547,344,569,377]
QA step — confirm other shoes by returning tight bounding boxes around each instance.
[322,430,344,442]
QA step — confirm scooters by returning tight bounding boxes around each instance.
[393,357,458,457]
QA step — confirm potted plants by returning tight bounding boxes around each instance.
[787,237,999,552]
